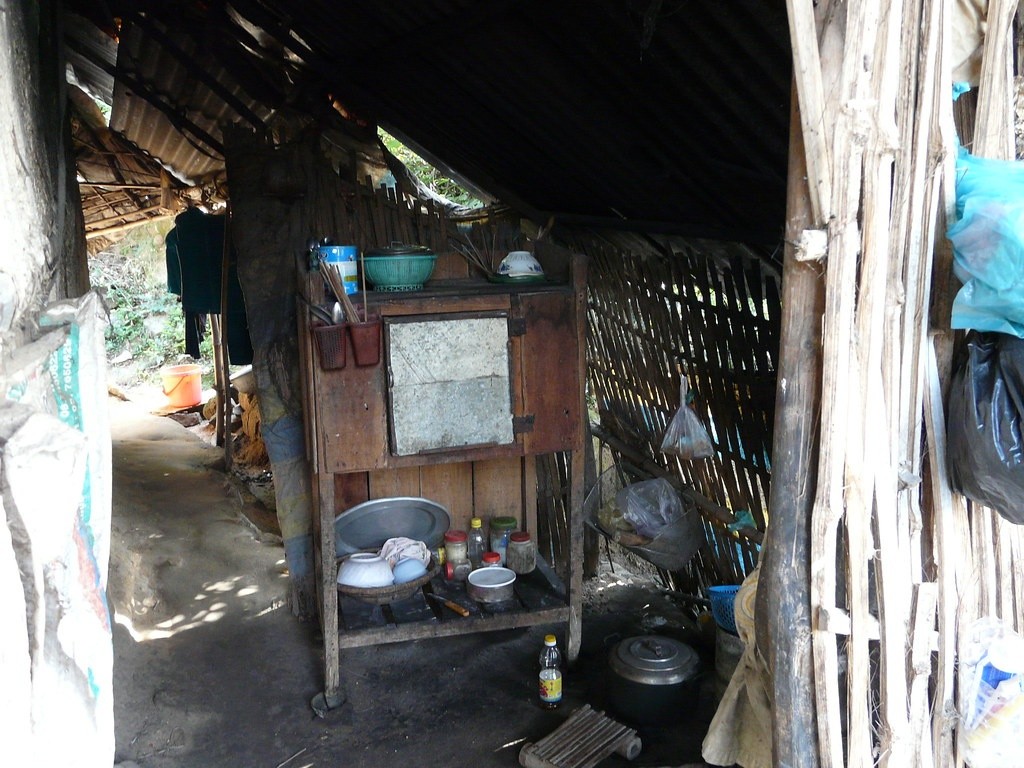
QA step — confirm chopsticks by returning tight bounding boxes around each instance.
[450,229,495,276]
[318,252,367,323]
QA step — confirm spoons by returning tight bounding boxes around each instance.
[332,302,344,324]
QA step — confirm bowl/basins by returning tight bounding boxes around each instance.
[392,557,427,585]
[496,251,544,275]
[336,552,395,588]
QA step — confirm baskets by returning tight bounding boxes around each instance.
[358,257,434,293]
[582,461,706,571]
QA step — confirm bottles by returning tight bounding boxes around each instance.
[507,532,536,574]
[481,552,503,567]
[490,516,517,567]
[538,635,563,710]
[466,517,486,569]
[445,558,472,581]
[445,530,467,561]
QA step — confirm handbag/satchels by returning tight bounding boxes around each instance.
[949,150,1024,343]
[659,375,715,461]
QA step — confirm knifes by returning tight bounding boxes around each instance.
[427,592,469,617]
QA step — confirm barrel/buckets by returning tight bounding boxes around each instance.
[159,364,202,407]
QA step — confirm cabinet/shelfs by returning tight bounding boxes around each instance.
[293,247,588,720]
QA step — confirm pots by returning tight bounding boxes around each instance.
[609,637,698,708]
